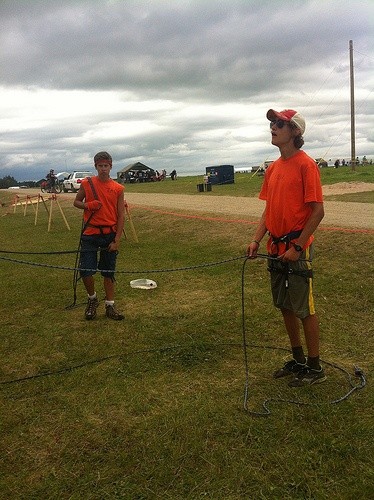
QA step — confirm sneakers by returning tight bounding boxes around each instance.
[287,365,327,388]
[273,357,308,379]
[84,295,99,320]
[105,304,125,320]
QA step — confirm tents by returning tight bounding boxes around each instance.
[117,162,154,183]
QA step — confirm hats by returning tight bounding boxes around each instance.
[266,108,306,135]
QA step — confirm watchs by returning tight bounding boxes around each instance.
[293,243,302,252]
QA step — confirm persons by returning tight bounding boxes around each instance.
[334,156,372,168]
[247,108,327,387]
[46,170,57,193]
[73,152,125,320]
[120,169,177,183]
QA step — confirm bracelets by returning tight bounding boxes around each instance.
[252,240,259,245]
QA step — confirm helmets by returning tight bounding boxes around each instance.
[50,168,54,172]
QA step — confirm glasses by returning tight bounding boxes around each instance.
[269,120,288,129]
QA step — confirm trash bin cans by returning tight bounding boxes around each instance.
[197,183,204,192]
[205,184,212,192]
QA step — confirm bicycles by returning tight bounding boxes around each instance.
[40,178,62,194]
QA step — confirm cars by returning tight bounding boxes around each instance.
[63,171,96,193]
